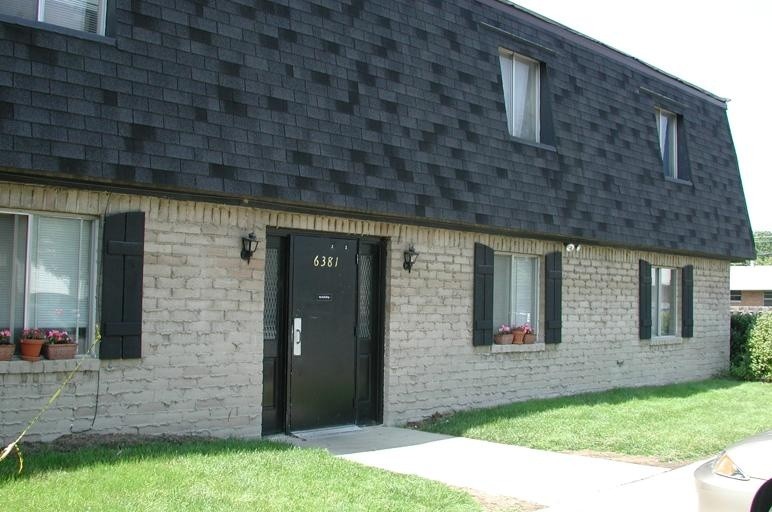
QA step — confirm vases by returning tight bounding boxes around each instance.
[1,337,78,360]
[494,331,537,344]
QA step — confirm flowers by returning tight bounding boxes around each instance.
[0,324,74,344]
[496,322,534,334]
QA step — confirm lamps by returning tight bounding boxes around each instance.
[563,240,582,253]
[240,233,261,264]
[402,244,419,274]
